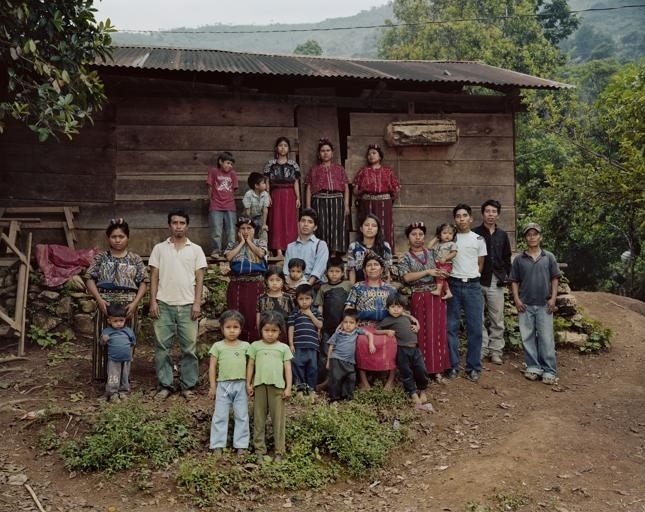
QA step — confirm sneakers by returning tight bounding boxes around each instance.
[491,355,503,365]
[542,375,555,385]
[524,372,537,381]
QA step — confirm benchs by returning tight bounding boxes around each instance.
[1,202,83,357]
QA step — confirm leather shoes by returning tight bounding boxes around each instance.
[446,369,458,378]
[464,371,479,382]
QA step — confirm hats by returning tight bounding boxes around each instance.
[521,222,541,235]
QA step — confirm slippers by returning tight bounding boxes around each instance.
[153,390,170,402]
[179,393,197,401]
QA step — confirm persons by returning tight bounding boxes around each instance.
[443,202,489,381]
[245,308,295,466]
[97,304,135,403]
[469,200,513,365]
[146,209,209,403]
[207,209,457,403]
[206,309,253,461]
[83,217,150,394]
[206,134,402,260]
[508,222,563,384]
